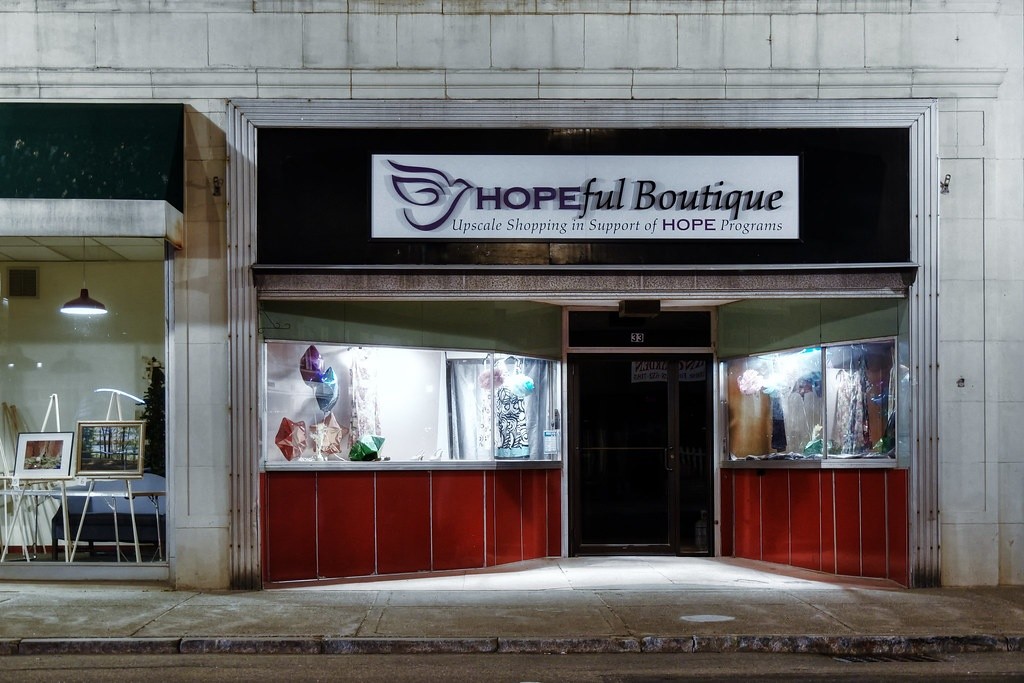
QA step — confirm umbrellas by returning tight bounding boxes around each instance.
[0,402,60,558]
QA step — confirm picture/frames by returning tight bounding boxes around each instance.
[13,432,74,480]
[75,420,146,479]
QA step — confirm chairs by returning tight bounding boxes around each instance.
[51,473,165,562]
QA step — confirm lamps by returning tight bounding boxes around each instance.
[60,238,107,315]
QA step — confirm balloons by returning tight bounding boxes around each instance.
[300,344,325,388]
[310,413,349,455]
[349,435,386,461]
[275,417,307,461]
[314,367,340,411]
